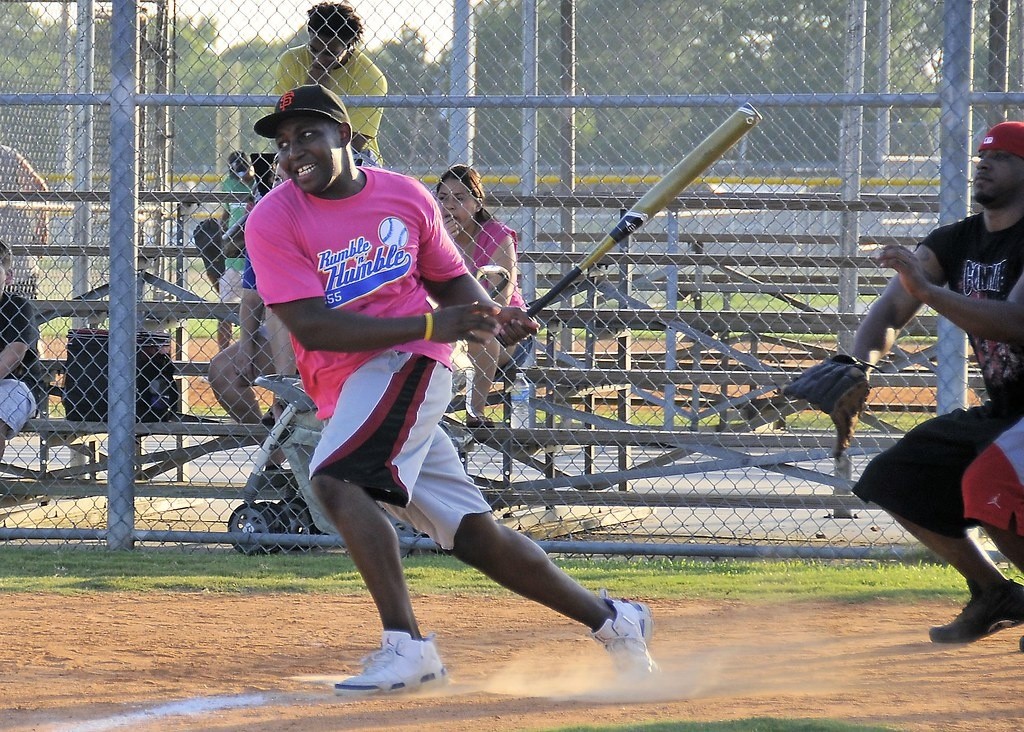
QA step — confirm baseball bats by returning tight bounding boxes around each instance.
[494,100,763,349]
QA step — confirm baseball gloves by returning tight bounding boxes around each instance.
[780,352,872,462]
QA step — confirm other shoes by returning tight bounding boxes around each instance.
[465,410,495,428]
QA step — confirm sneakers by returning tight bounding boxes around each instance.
[1019,636,1024,652]
[590,588,660,686]
[929,578,1024,644]
[335,630,447,697]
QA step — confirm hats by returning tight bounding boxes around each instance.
[254,85,350,140]
[978,121,1024,158]
[228,151,250,179]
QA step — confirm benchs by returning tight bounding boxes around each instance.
[0,186,983,511]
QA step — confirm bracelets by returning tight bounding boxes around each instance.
[424,313,433,342]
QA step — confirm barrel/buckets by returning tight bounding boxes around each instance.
[62,329,179,423]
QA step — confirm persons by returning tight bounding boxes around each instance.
[218,149,261,351]
[0,244,40,459]
[209,251,300,466]
[193,3,387,278]
[0,144,50,295]
[432,164,532,428]
[783,121,1024,649]
[247,86,658,694]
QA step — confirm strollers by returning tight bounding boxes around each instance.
[225,263,509,556]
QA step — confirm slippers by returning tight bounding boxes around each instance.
[261,403,284,426]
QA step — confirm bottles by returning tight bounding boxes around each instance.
[510,373,530,429]
